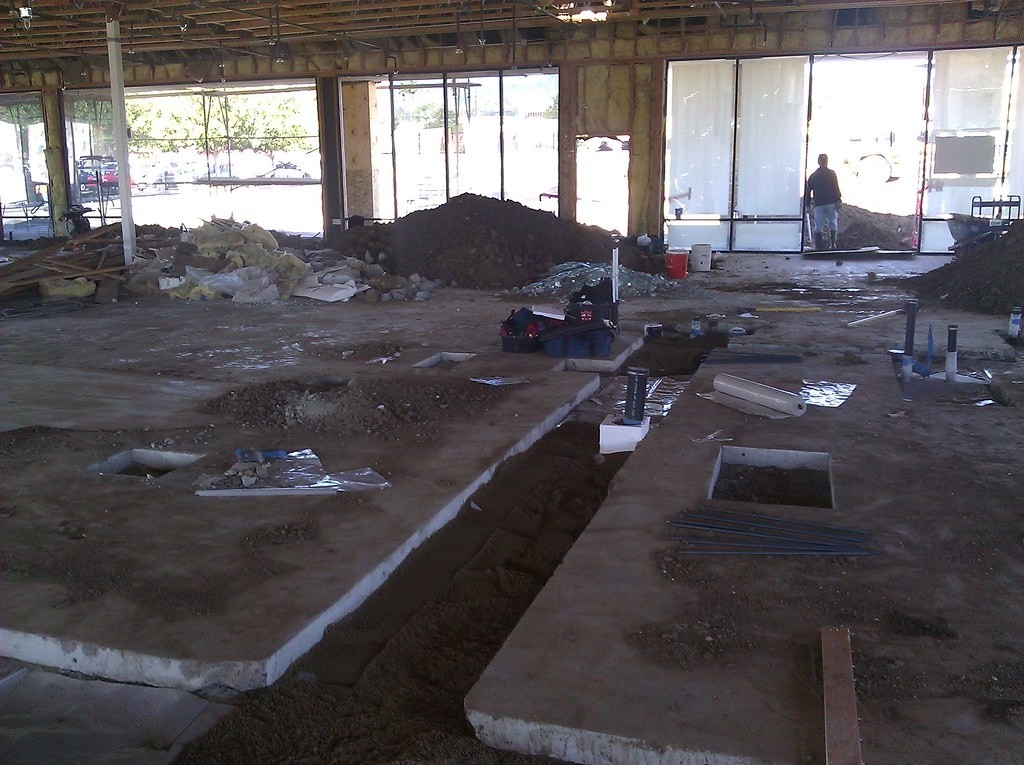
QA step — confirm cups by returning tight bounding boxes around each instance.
[622,367,648,425]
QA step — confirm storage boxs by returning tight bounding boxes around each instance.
[499,298,620,359]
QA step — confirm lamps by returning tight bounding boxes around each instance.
[18,6,33,29]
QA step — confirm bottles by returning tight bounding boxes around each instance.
[690,316,702,336]
[1008,307,1021,337]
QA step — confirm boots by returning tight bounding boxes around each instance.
[814,232,823,250]
[828,230,837,249]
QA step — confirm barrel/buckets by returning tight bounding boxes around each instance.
[666,249,689,279]
[692,243,712,271]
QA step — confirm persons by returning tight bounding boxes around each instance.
[806,154,843,249]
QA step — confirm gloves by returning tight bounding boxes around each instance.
[834,201,843,212]
[807,207,815,218]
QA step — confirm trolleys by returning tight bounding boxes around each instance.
[946,212,1010,261]
